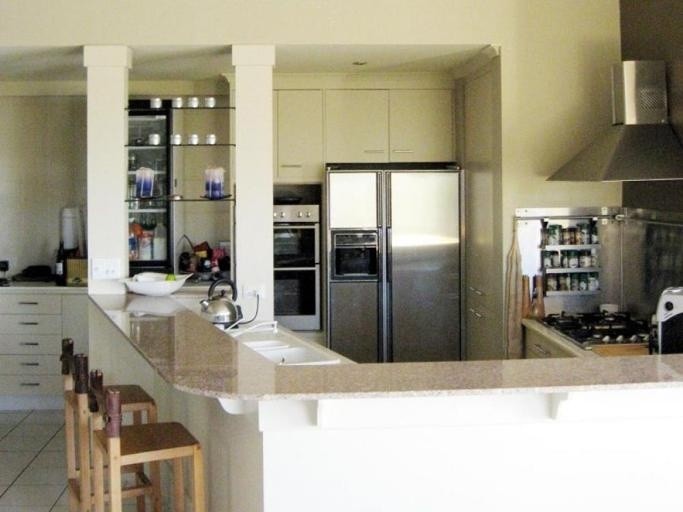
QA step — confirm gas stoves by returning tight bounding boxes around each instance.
[537,310,649,350]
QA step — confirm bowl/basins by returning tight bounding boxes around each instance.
[120,271,193,297]
[599,302,618,321]
[121,296,195,318]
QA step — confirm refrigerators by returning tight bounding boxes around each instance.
[320,163,467,363]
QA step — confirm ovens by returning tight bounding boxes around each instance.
[273,204,320,330]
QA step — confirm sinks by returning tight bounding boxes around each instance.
[242,340,308,350]
[257,347,340,367]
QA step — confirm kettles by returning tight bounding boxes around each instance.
[199,279,243,330]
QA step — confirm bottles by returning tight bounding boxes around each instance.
[127,213,166,260]
[61,207,79,249]
[146,97,216,147]
[0,260,13,287]
[650,314,657,348]
[522,217,600,318]
[54,240,67,287]
[128,136,165,171]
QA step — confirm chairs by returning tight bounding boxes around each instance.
[58,334,164,512]
[83,368,208,511]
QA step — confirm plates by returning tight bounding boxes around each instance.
[130,194,163,201]
[199,195,233,200]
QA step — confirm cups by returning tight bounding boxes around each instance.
[175,242,229,282]
[204,165,224,197]
[135,166,154,197]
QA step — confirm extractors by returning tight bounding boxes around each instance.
[542,60,683,182]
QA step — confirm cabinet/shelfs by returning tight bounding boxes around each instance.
[0,277,61,415]
[514,320,587,358]
[537,212,603,300]
[120,99,235,206]
[317,68,461,170]
[215,70,325,190]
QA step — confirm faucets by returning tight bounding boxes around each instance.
[224,318,279,338]
